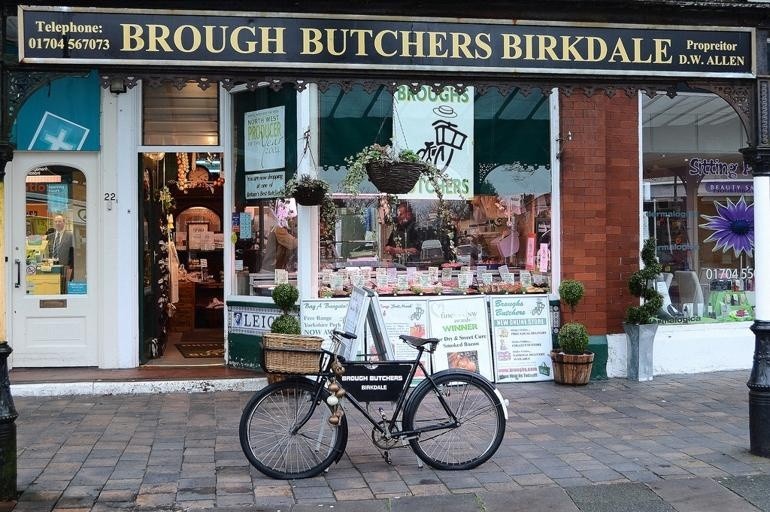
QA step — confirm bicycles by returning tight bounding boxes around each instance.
[238,327,509,477]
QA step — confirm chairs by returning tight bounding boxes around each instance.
[663,270,707,315]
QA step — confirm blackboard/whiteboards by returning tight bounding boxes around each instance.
[338,285,367,361]
[363,286,395,362]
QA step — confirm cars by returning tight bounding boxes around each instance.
[345,240,486,266]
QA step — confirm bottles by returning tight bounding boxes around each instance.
[683,305,688,318]
[708,303,713,317]
[718,277,741,291]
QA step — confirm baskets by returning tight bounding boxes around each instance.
[257,341,325,377]
[261,332,324,372]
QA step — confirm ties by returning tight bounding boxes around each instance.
[53,233,60,259]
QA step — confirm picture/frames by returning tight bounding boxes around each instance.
[186,220,210,252]
[299,294,557,389]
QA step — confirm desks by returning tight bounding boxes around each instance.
[709,290,756,309]
[248,266,525,292]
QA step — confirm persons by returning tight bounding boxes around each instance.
[385,200,424,262]
[260,214,298,274]
[47,214,74,281]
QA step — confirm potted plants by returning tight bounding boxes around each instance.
[281,142,448,205]
[551,278,595,386]
[623,237,666,385]
[265,283,306,395]
